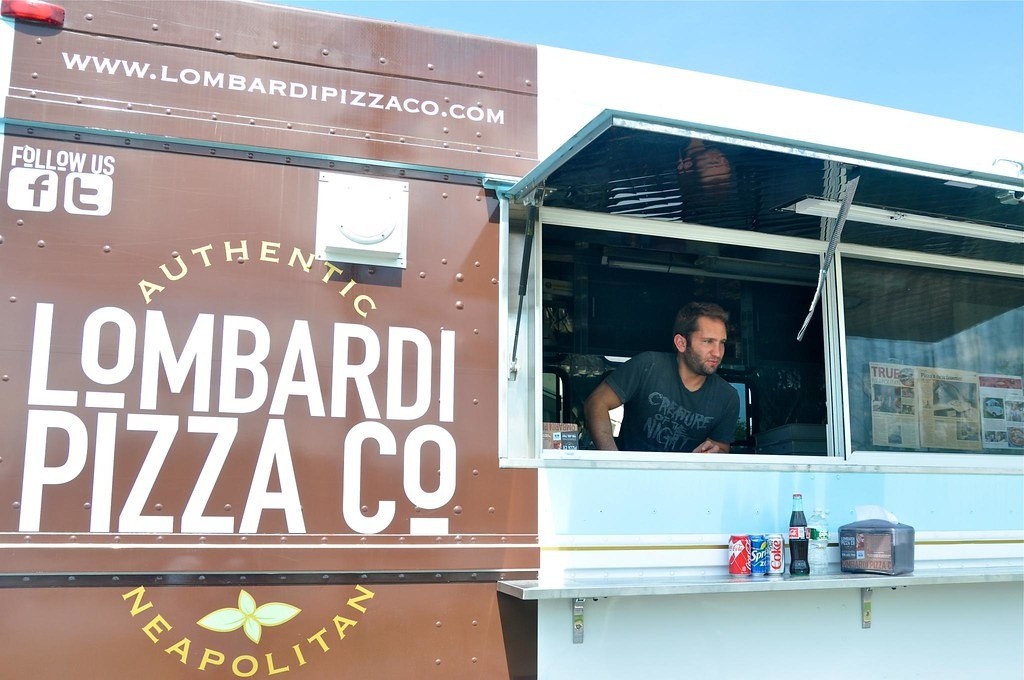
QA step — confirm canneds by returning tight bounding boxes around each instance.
[728,533,786,576]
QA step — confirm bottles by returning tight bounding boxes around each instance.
[789,494,810,575]
[808,507,829,575]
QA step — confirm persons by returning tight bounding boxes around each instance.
[677,136,735,197]
[583,302,741,453]
